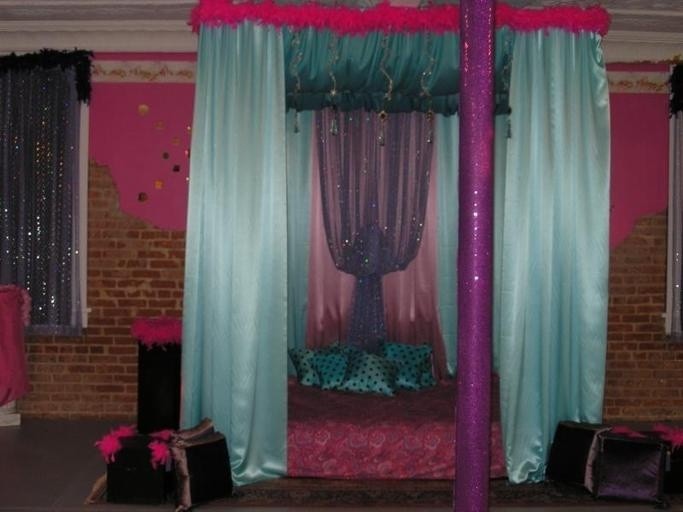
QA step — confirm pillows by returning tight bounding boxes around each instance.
[289,342,439,396]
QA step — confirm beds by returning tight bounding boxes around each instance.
[287,376,502,480]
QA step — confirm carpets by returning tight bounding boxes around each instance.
[80,470,682,508]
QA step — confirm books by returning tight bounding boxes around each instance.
[176,417,216,443]
